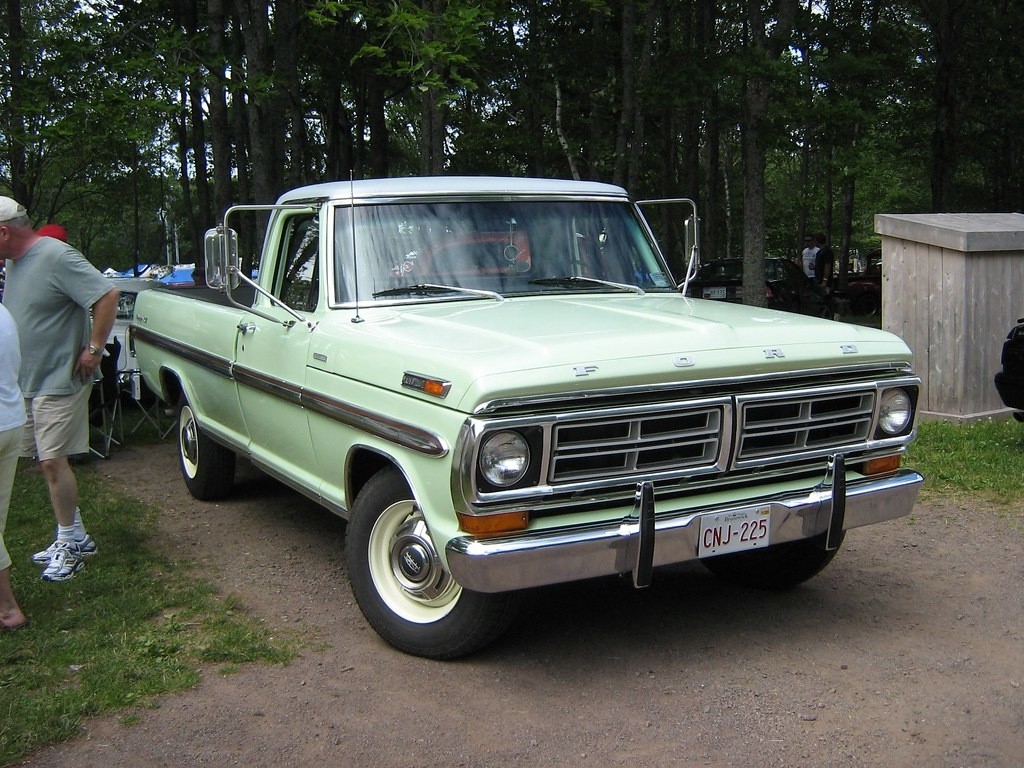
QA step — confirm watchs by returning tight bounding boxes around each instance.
[86,342,104,356]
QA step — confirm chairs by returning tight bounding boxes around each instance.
[84,334,178,460]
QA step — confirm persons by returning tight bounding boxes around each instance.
[0,298,29,635]
[0,195,119,582]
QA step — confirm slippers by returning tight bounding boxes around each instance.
[0,617,32,632]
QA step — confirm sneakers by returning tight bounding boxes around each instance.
[29,534,98,564]
[41,542,85,581]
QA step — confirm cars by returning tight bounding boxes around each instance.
[681,257,835,321]
[88,277,169,402]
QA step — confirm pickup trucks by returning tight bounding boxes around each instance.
[127,165,923,664]
[831,248,881,318]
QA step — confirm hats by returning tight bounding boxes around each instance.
[0,194,28,222]
[33,223,68,243]
